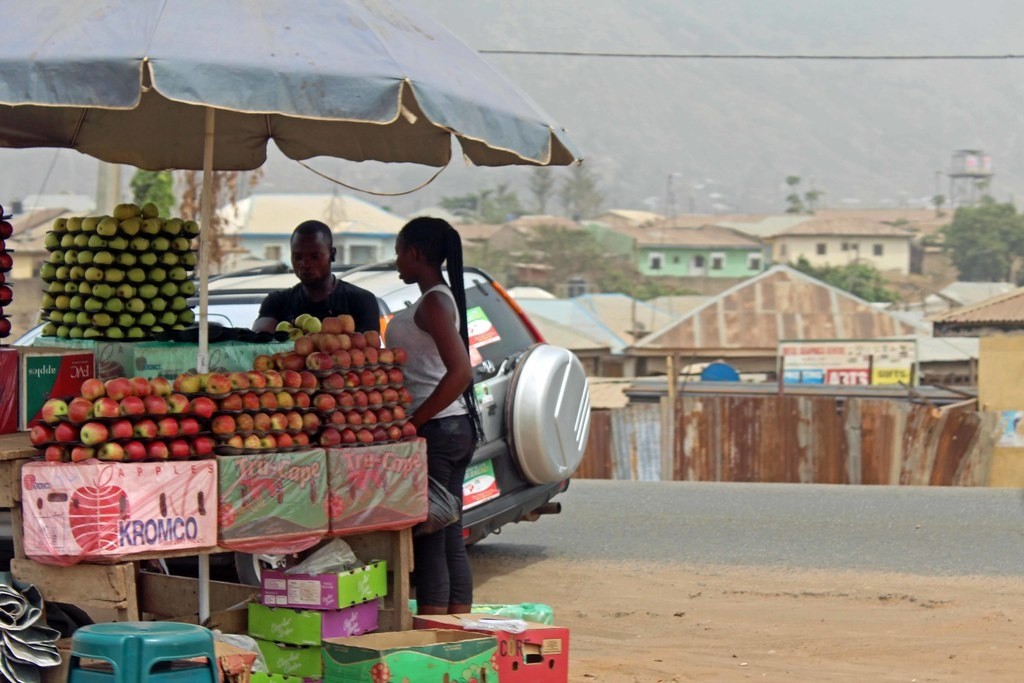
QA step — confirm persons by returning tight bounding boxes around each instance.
[385,216,488,615]
[252,220,380,335]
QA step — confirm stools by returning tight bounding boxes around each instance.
[69,620,220,683]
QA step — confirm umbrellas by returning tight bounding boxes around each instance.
[0,0,584,630]
[0,579,95,683]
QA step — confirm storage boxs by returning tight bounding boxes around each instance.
[0,332,570,683]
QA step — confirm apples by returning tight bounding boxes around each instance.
[0,205,14,334]
[41,202,200,337]
[29,314,415,461]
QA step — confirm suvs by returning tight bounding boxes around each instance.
[10,256,592,586]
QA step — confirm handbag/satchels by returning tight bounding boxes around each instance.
[415,476,462,538]
